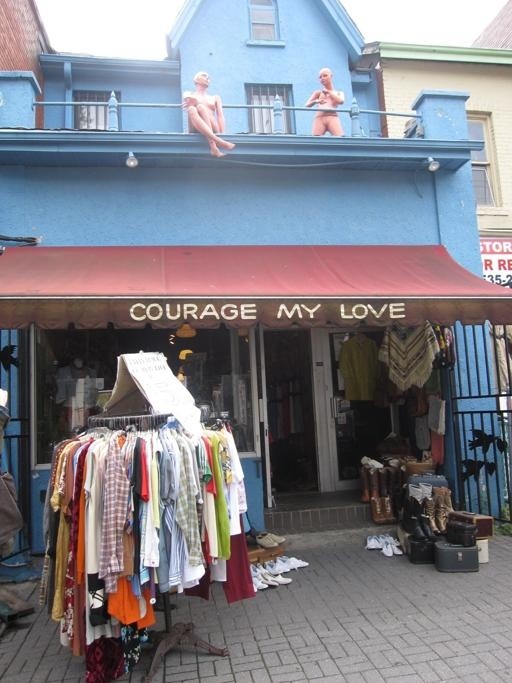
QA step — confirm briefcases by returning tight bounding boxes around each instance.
[434,510,494,572]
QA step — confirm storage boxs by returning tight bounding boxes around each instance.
[397,473,494,572]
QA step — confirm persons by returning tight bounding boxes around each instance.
[37,355,66,422]
[181,68,236,160]
[306,65,345,136]
[55,357,99,433]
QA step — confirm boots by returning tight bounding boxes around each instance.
[361,466,396,524]
[404,482,454,541]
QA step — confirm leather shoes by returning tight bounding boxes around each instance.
[256,532,285,548]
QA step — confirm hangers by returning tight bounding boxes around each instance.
[75,415,222,438]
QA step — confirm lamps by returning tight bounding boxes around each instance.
[413,156,440,197]
[176,322,197,338]
[125,152,139,168]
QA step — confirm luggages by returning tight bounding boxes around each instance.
[409,473,448,487]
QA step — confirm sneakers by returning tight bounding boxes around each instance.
[253,571,292,589]
[367,534,403,556]
[251,556,309,576]
